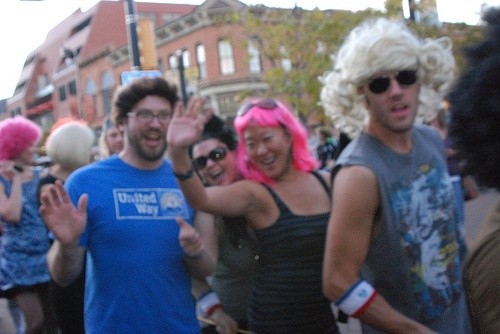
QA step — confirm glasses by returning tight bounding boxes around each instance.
[237,97,277,117]
[193,147,226,169]
[369,70,418,94]
[138,110,170,119]
